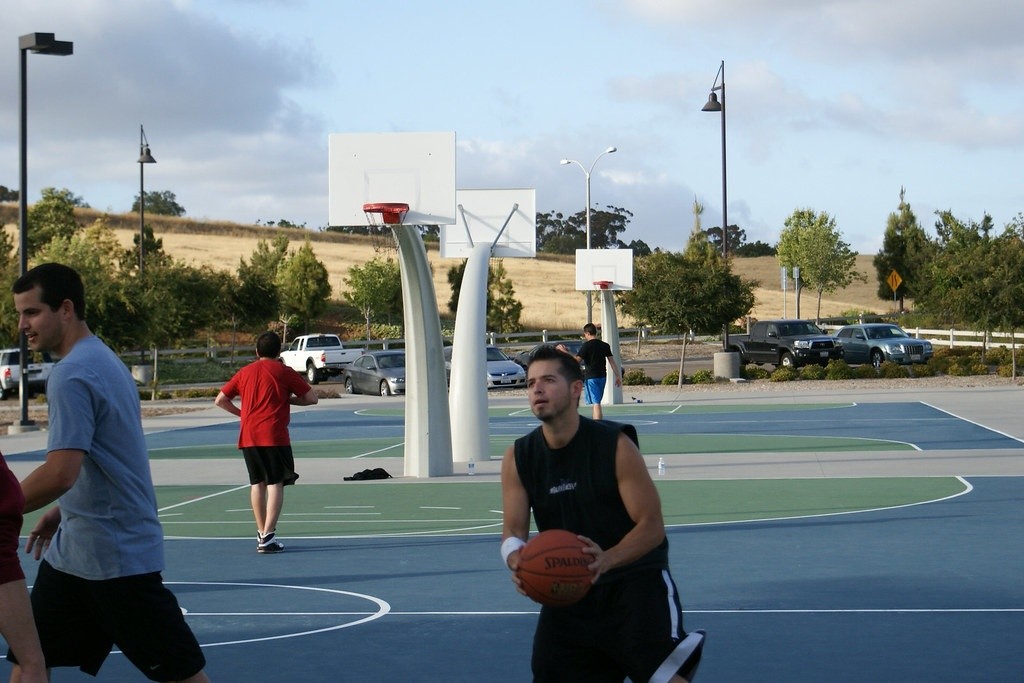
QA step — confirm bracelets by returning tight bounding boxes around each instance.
[500,536,527,570]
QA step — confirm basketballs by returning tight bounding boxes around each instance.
[517,529,595,607]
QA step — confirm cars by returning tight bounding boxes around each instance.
[342,348,405,398]
[443,346,527,391]
[829,323,933,369]
[513,339,625,380]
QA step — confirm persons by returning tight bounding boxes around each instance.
[214,332,318,554]
[5,261,210,683]
[499,344,706,683]
[555,323,622,420]
[0,451,49,683]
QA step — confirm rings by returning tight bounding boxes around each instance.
[37,536,44,539]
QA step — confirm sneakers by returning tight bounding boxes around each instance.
[257,530,285,553]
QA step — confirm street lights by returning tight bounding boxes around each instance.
[559,145,617,324]
[17,32,74,418]
[701,58,729,352]
[135,124,158,276]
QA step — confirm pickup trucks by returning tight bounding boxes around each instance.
[0,347,56,401]
[278,333,365,385]
[722,319,842,371]
